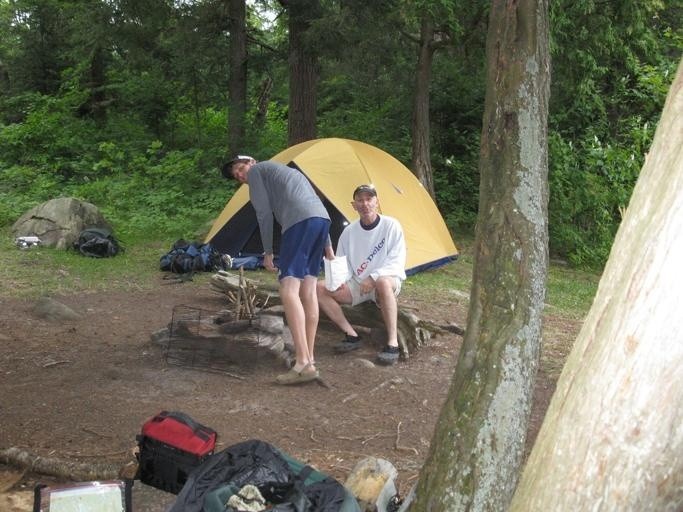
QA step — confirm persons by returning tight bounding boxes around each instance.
[314,184,407,364]
[222,153,338,386]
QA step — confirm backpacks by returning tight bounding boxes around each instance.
[167,437,361,512]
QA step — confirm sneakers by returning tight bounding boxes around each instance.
[374,343,401,365]
[332,335,366,355]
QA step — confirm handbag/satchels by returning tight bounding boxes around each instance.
[133,407,217,497]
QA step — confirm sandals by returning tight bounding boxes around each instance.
[273,357,322,385]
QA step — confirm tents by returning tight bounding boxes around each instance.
[200,135,461,278]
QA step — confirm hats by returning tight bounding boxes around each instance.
[219,154,256,180]
[352,184,378,201]
